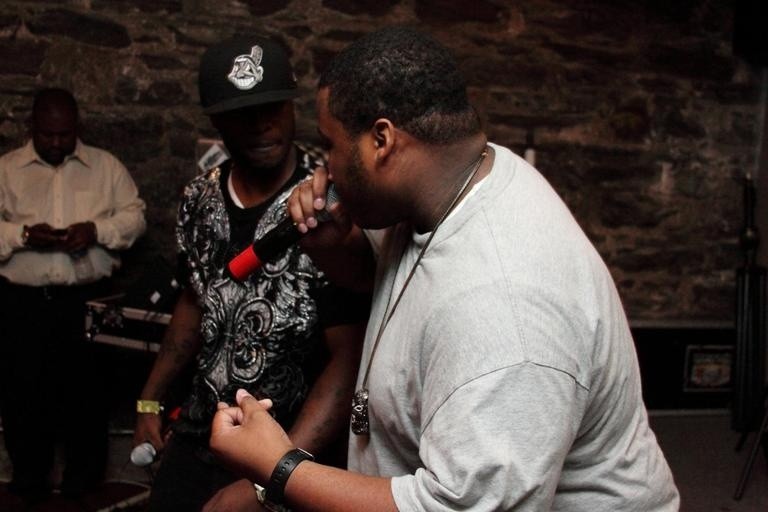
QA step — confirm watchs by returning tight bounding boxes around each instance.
[251,448,312,511]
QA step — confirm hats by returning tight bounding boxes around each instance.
[198,35,299,114]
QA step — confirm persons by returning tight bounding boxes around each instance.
[208,25,684,512]
[133,28,378,512]
[0,86,146,498]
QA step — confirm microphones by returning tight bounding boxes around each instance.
[225,184,340,280]
[130,425,173,467]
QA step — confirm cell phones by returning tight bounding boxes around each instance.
[50,228,68,236]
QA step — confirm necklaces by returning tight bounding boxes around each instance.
[347,146,490,436]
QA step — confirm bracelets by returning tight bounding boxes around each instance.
[134,398,163,415]
[22,224,29,246]
